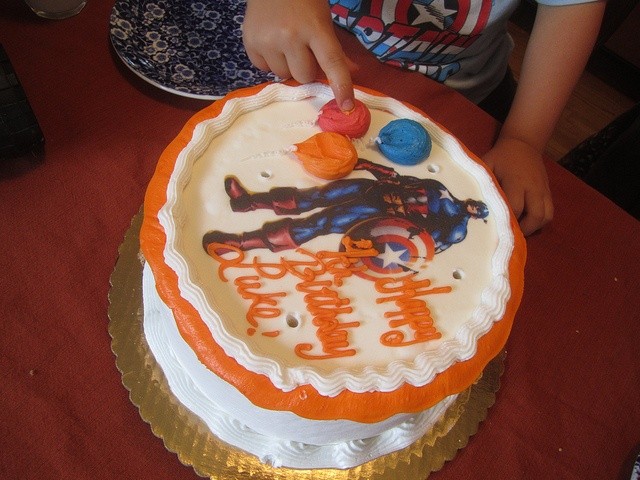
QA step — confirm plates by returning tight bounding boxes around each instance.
[106,201,509,480]
[109,0,289,102]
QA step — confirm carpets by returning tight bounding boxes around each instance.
[556,103,640,235]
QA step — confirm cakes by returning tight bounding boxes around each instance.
[140,78,527,470]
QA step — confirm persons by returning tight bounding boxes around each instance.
[241,0,607,238]
[203,157,489,258]
